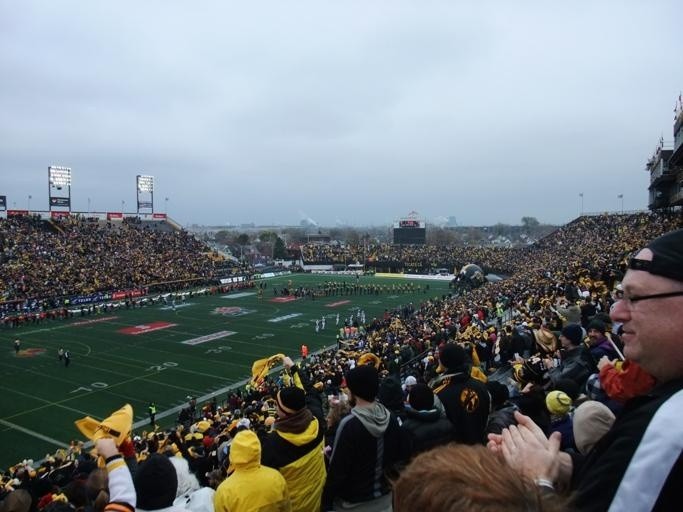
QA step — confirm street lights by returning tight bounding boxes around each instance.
[615,192,625,215]
[577,191,585,213]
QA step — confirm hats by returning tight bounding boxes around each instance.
[534,328,557,353]
[409,383,434,411]
[585,319,606,335]
[627,228,682,283]
[560,324,583,346]
[275,387,306,416]
[133,454,178,511]
[345,365,378,403]
[545,389,572,415]
[439,343,466,369]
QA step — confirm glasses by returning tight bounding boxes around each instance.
[613,287,683,304]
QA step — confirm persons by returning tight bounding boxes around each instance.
[0,208,682,512]
